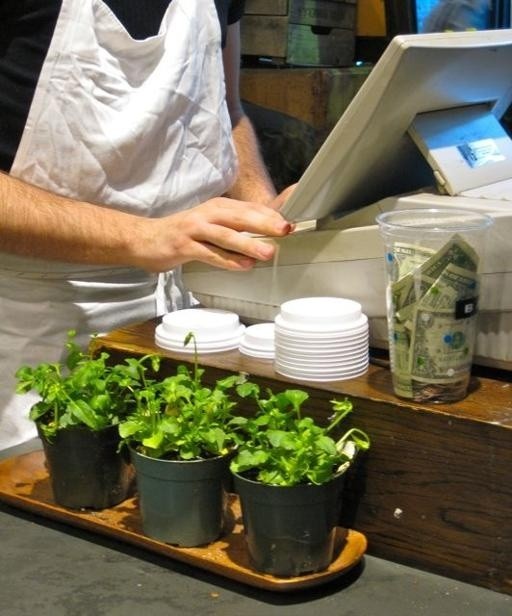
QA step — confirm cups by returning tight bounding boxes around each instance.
[375,207,494,405]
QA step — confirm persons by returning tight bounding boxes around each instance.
[0,0,299,450]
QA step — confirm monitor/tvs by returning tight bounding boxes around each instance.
[278,27,512,225]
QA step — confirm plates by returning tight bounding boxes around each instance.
[238,323,277,359]
[1,450,367,593]
[154,307,246,354]
[273,297,370,384]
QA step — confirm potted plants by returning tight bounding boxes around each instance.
[118,332,251,548]
[229,381,371,576]
[14,329,160,510]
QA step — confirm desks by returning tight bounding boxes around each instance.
[92,303,512,597]
